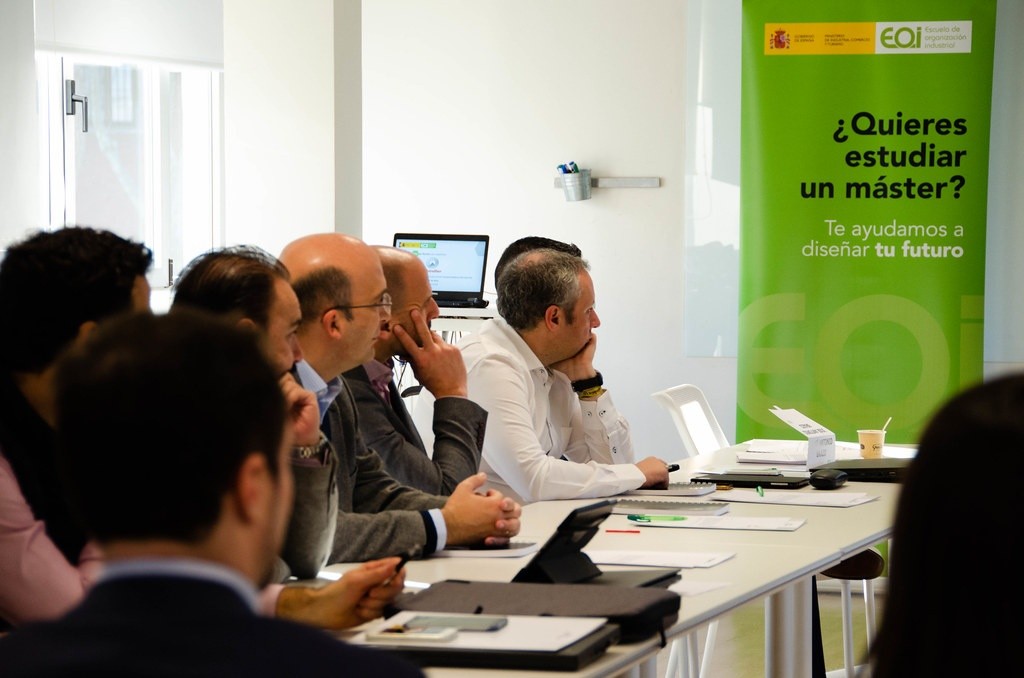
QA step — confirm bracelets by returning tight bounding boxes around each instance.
[571,372,603,393]
[288,430,327,459]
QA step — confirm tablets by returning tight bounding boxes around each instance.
[512,499,618,583]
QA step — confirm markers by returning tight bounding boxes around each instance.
[668,464,680,472]
[557,162,579,173]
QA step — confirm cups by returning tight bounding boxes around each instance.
[857,429,887,459]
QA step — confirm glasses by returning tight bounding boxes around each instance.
[320,293,392,323]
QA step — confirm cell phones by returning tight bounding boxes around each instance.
[367,629,459,641]
[405,616,508,632]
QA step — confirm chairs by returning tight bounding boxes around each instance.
[652,384,887,678]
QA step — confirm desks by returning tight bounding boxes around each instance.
[320,441,917,678]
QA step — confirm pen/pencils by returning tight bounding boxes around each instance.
[382,543,420,586]
[756,485,764,497]
[627,514,687,522]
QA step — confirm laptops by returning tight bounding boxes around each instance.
[689,474,809,489]
[810,458,913,482]
[393,233,488,310]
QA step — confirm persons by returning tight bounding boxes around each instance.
[414,237,670,505]
[164,244,340,583]
[323,244,491,508]
[860,371,1023,677]
[0,226,407,628]
[276,233,522,564]
[0,309,424,678]
